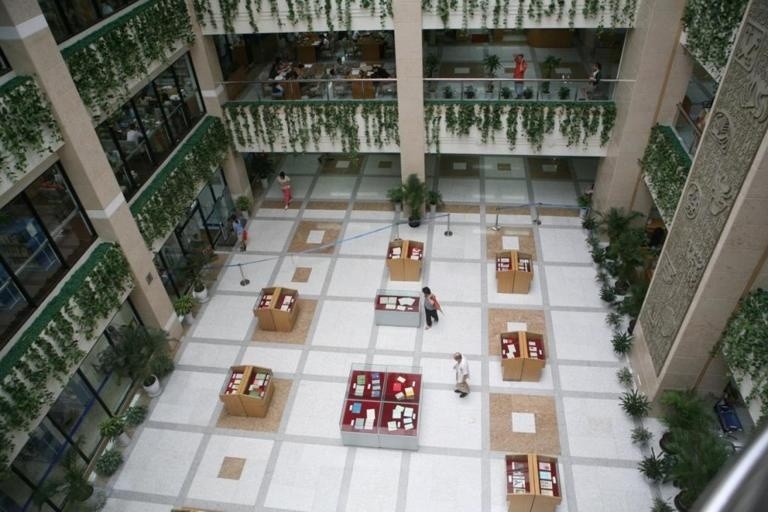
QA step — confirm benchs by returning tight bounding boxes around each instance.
[100,103,188,165]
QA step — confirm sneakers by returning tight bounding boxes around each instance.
[455,389,467,398]
[424,320,437,330]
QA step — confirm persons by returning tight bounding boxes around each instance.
[588,61,602,97]
[229,31,394,93]
[513,52,527,99]
[422,286,438,330]
[278,172,292,209]
[231,214,246,252]
[95,80,193,191]
[452,351,469,397]
[41,172,65,196]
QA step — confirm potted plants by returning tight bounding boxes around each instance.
[423,53,441,99]
[256,167,271,190]
[186,269,210,304]
[385,173,445,227]
[235,197,250,221]
[439,54,569,99]
[173,297,196,324]
[36,319,181,512]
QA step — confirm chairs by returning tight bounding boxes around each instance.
[259,31,395,100]
